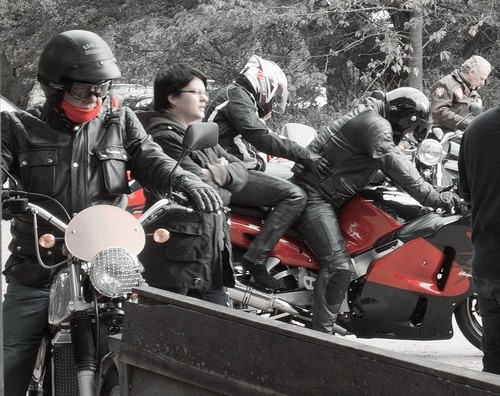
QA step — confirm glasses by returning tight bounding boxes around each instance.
[170,88,211,97]
[56,78,112,100]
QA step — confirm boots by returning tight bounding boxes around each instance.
[311,254,351,334]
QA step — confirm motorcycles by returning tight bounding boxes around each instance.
[262,100,484,208]
[103,89,484,351]
[0,123,231,396]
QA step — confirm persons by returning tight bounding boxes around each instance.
[0,29,222,396]
[458,106,500,376]
[288,87,461,336]
[202,54,332,290]
[141,64,248,308]
[426,56,491,142]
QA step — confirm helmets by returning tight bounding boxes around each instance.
[236,55,287,121]
[383,87,432,146]
[39,29,121,81]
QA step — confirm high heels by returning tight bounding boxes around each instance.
[240,257,282,290]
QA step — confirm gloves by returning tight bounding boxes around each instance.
[306,149,333,181]
[171,175,224,212]
[433,192,464,214]
[2,188,13,220]
